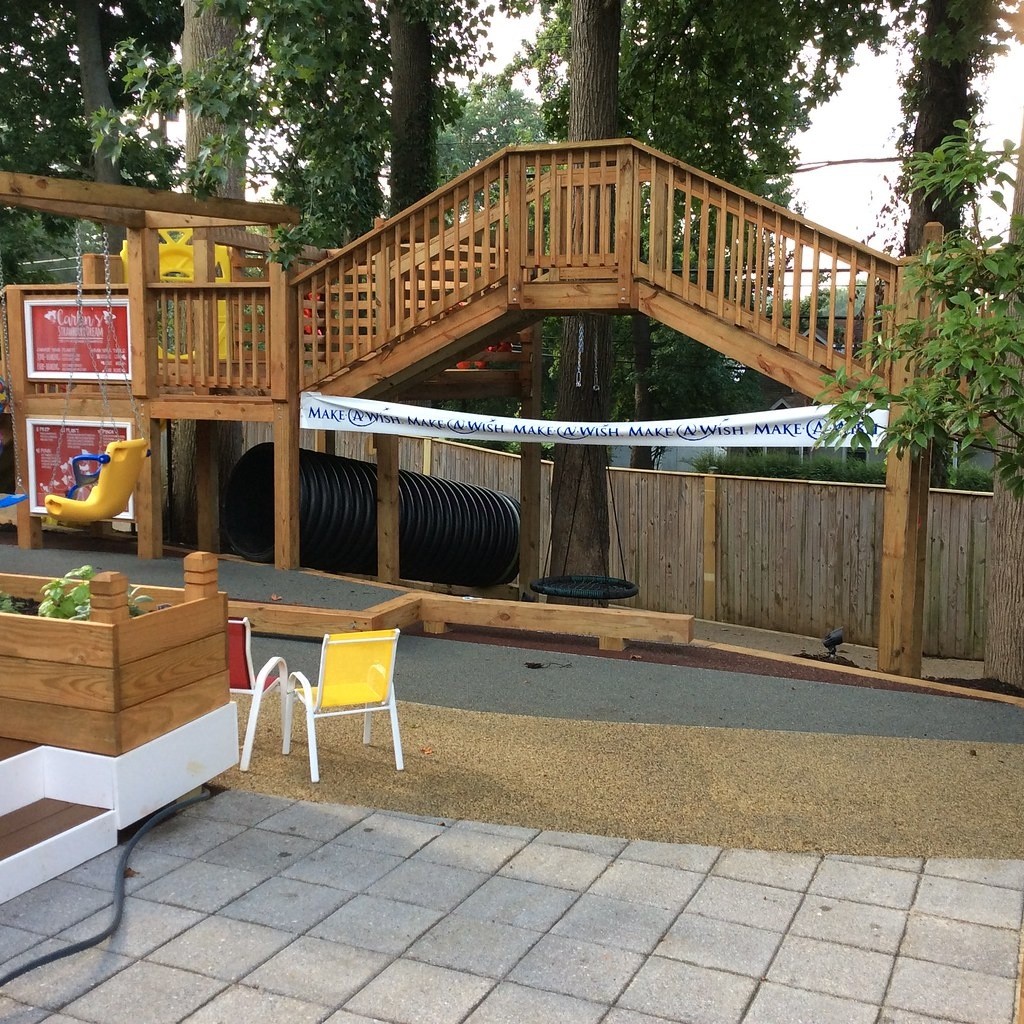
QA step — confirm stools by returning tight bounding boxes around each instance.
[43,438,149,522]
[0,492,28,510]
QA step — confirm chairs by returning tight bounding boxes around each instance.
[285,626,404,786]
[228,617,289,776]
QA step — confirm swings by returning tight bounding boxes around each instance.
[45,217,152,523]
